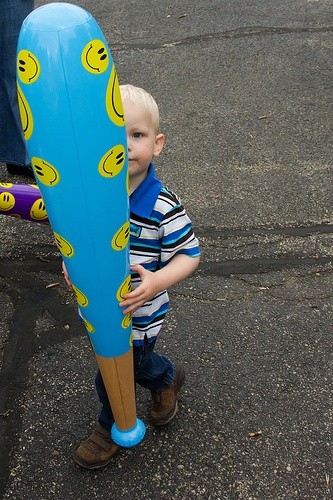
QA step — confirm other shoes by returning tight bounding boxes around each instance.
[6,163,34,180]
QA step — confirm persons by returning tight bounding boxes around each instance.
[0,0,36,180]
[62,84,202,470]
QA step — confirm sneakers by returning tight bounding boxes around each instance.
[73,423,119,470]
[150,364,186,426]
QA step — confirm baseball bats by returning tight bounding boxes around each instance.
[0,182,51,225]
[15,2,147,448]
